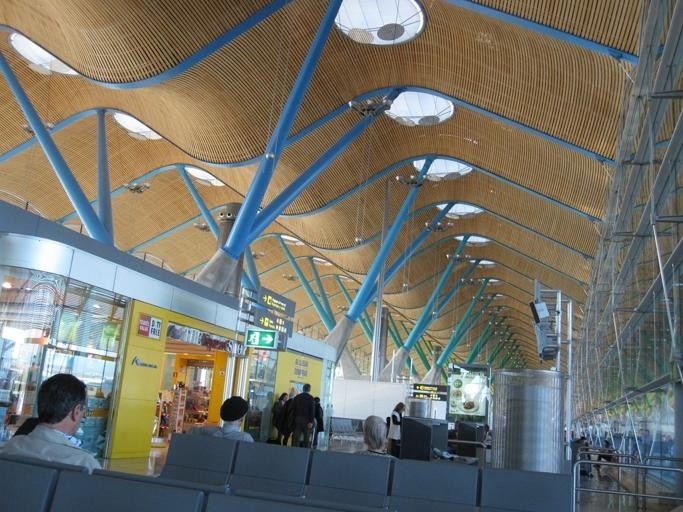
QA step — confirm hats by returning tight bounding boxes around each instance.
[219,396,248,421]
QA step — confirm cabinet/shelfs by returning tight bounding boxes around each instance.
[155,388,210,438]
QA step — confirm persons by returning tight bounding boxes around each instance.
[210,395,255,443]
[631,428,673,465]
[387,403,406,457]
[353,415,399,459]
[448,420,461,451]
[481,424,493,449]
[577,436,594,479]
[0,373,103,474]
[271,382,325,449]
[594,439,614,469]
